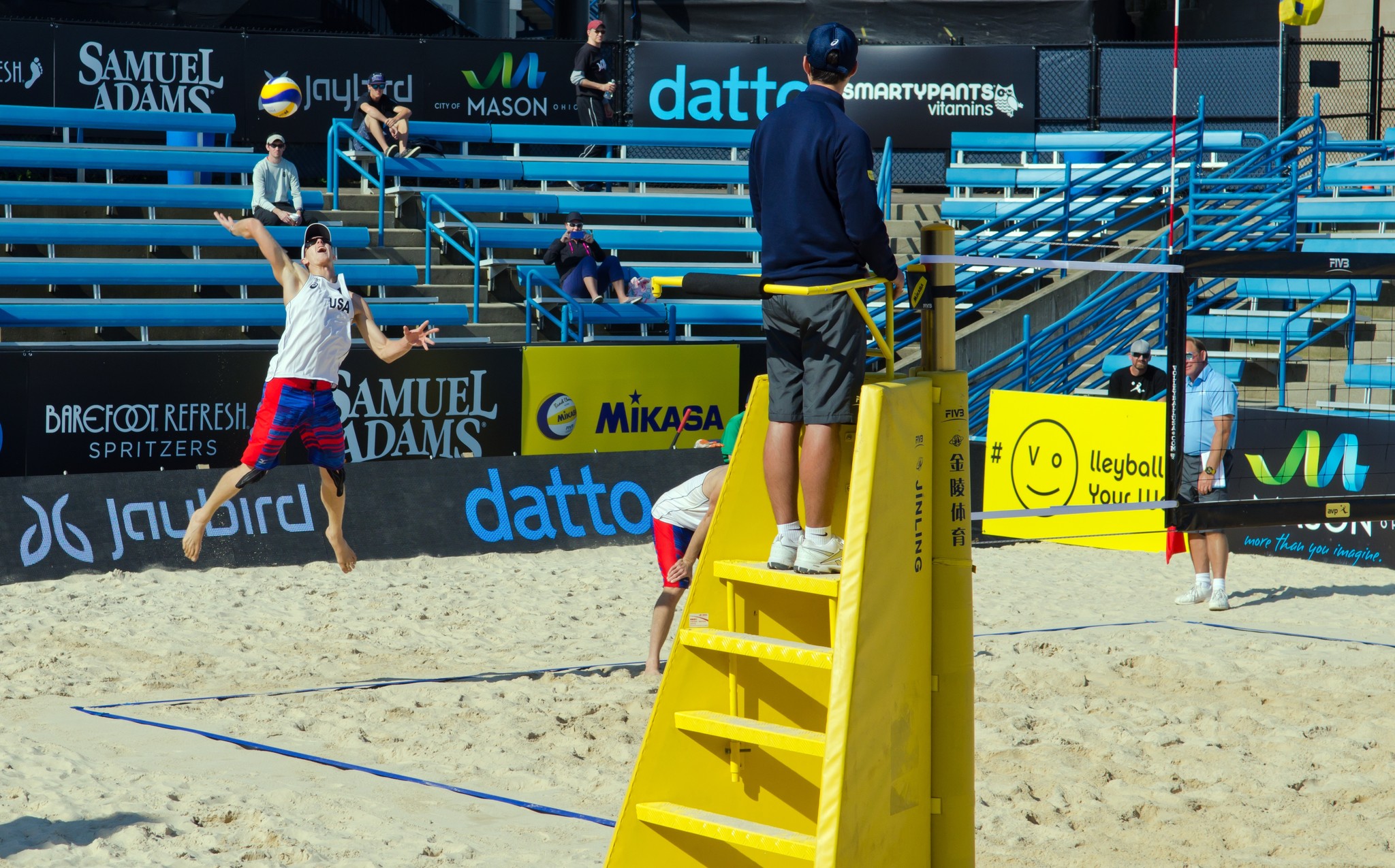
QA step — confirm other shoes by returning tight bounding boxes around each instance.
[622,295,643,304]
[385,144,399,157]
[592,295,604,303]
[399,146,421,157]
[584,187,606,192]
[568,180,583,191]
[627,277,657,303]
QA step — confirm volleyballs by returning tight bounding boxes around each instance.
[260,76,302,118]
[535,392,577,441]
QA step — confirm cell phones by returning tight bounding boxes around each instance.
[570,231,584,239]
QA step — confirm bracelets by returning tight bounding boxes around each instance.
[229,225,234,234]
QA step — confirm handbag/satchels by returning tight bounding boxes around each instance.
[407,137,446,158]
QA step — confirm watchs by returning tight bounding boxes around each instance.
[1204,466,1216,475]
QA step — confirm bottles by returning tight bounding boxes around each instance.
[603,79,616,99]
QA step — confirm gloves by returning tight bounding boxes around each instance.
[694,438,724,449]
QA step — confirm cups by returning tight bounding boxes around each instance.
[289,213,299,226]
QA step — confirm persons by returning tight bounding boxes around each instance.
[566,20,617,191]
[182,212,440,574]
[1109,338,1237,611]
[351,72,421,157]
[543,211,644,303]
[642,391,748,678]
[749,22,903,576]
[252,134,318,226]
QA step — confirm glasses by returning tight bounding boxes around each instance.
[594,30,606,34]
[268,143,284,148]
[305,237,332,247]
[370,84,385,89]
[567,222,583,227]
[1186,352,1193,360]
[1131,352,1150,358]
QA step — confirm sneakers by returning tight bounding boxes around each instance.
[794,535,842,573]
[768,533,797,570]
[1175,584,1213,606]
[1209,589,1230,610]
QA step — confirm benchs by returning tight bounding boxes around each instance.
[0,104,1395,416]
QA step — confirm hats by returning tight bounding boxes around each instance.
[301,223,335,259]
[587,20,606,30]
[1131,339,1150,357]
[368,72,387,84]
[267,134,285,144]
[808,23,858,77]
[565,212,583,223]
